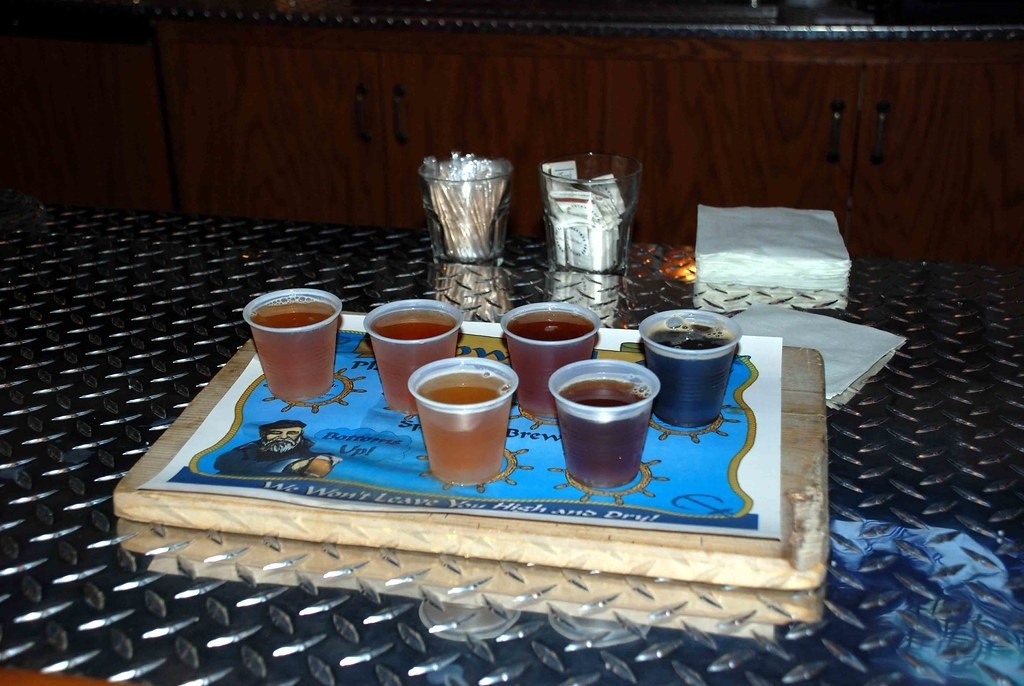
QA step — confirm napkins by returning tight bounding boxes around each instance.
[692,204,906,400]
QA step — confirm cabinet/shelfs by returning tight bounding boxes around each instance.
[600,36,1024,264]
[156,19,601,238]
[0,41,176,211]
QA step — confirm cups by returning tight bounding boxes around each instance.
[500,301,600,417]
[363,298,463,415]
[408,356,519,486]
[639,309,742,428]
[539,152,643,276]
[548,358,661,488]
[242,288,342,402]
[416,156,514,266]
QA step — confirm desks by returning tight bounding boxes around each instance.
[0,198,1024,686]
